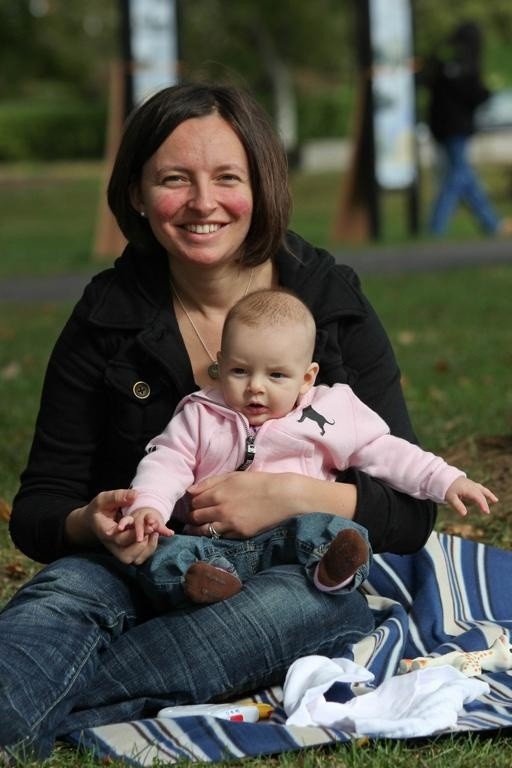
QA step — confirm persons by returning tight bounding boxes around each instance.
[0,80,439,767]
[105,287,499,608]
[424,20,512,242]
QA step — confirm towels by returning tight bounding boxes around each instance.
[66,528,512,766]
[266,651,490,740]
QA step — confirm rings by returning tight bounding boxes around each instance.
[208,522,222,539]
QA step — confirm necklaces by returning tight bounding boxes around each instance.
[165,259,255,380]
